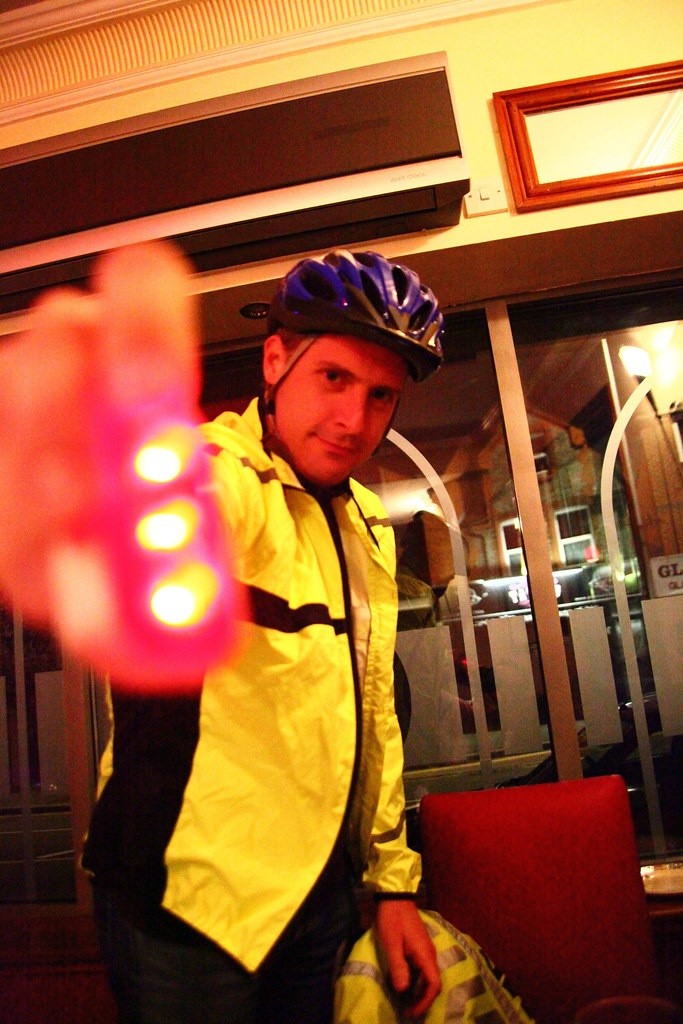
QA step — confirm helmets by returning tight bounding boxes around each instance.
[265,250,444,383]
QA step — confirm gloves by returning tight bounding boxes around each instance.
[378,900,441,1018]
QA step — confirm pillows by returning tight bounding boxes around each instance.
[420,775,660,1024]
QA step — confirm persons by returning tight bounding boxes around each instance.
[0,240,443,1023]
[393,511,473,849]
